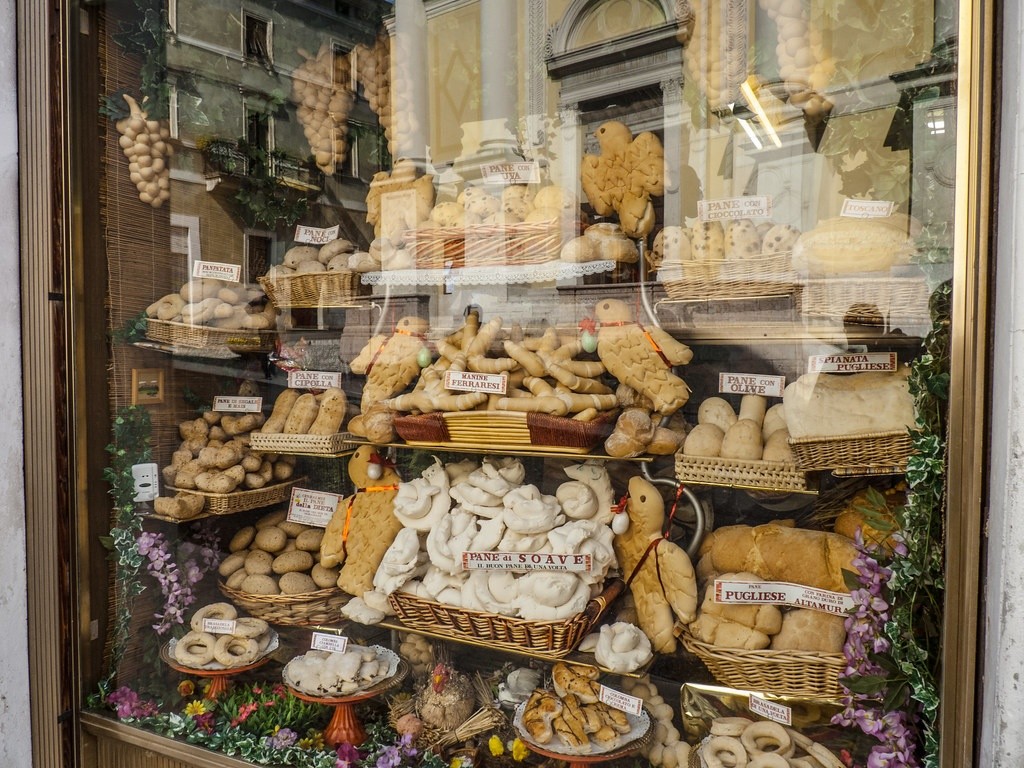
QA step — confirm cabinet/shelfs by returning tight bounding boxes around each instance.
[130,259,935,744]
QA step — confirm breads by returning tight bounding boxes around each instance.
[579,119,665,237]
[682,522,873,654]
[651,212,926,281]
[320,445,408,597]
[219,510,342,595]
[261,239,413,278]
[413,183,566,226]
[592,299,694,414]
[260,387,347,436]
[383,309,620,422]
[144,277,281,331]
[684,394,796,464]
[562,222,640,263]
[346,316,434,442]
[613,476,698,655]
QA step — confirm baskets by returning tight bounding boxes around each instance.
[144,218,931,768]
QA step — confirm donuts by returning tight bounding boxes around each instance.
[175,601,268,668]
